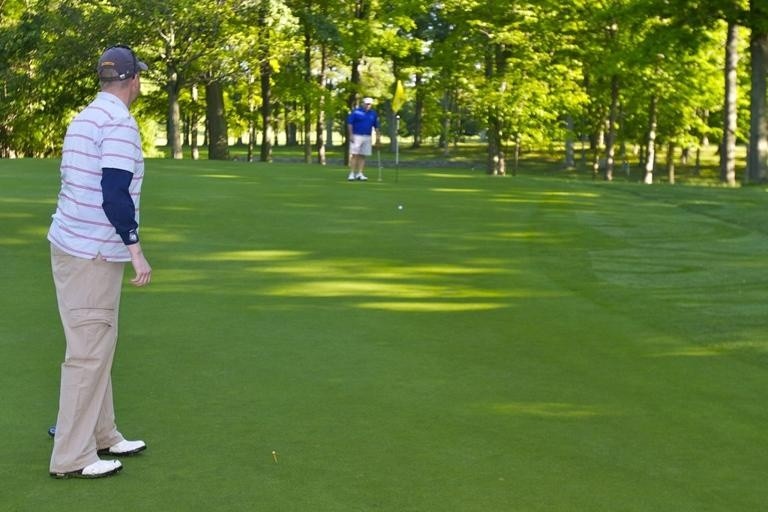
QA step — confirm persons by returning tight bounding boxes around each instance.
[346,97,381,182]
[44,43,153,478]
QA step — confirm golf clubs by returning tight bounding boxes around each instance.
[377,150,381,181]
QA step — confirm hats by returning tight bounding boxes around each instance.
[363,98,373,104]
[97,45,148,81]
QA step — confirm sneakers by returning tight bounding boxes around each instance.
[347,173,367,181]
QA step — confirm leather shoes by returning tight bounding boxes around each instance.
[97,440,146,456]
[50,460,123,479]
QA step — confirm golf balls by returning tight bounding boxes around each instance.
[397,205,402,210]
